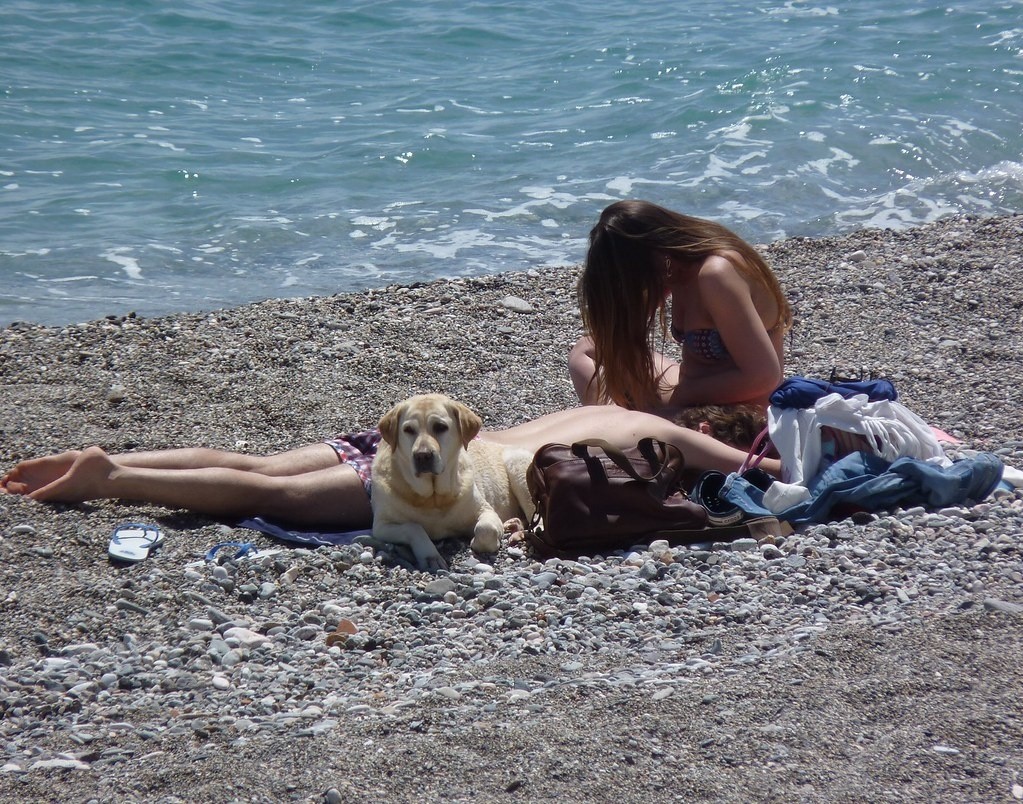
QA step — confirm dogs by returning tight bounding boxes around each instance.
[369,393,541,570]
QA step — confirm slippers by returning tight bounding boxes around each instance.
[109,523,165,562]
[187,544,282,571]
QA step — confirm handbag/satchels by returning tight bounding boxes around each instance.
[526,436,708,556]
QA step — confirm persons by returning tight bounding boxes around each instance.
[2,406,782,532]
[564,198,795,422]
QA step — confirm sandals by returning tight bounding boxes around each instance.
[741,467,776,494]
[691,470,742,524]
